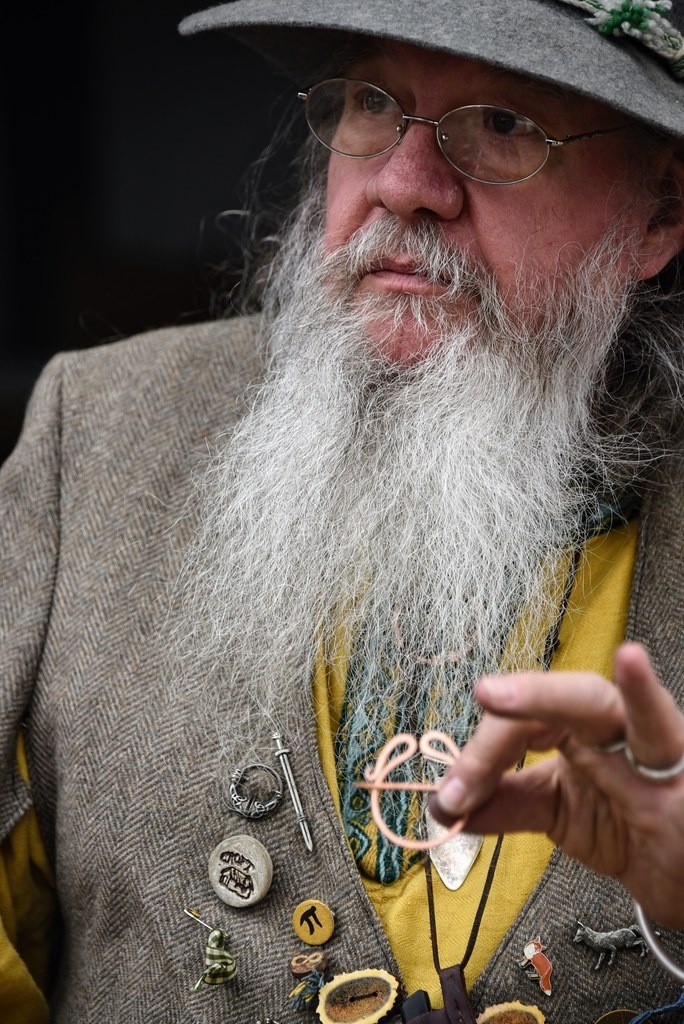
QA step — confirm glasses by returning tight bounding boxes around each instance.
[295,77,645,185]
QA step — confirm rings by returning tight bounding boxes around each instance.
[616,714,684,788]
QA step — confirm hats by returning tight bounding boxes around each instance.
[180,0,684,142]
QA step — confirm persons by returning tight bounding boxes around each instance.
[1,0,684,1024]
